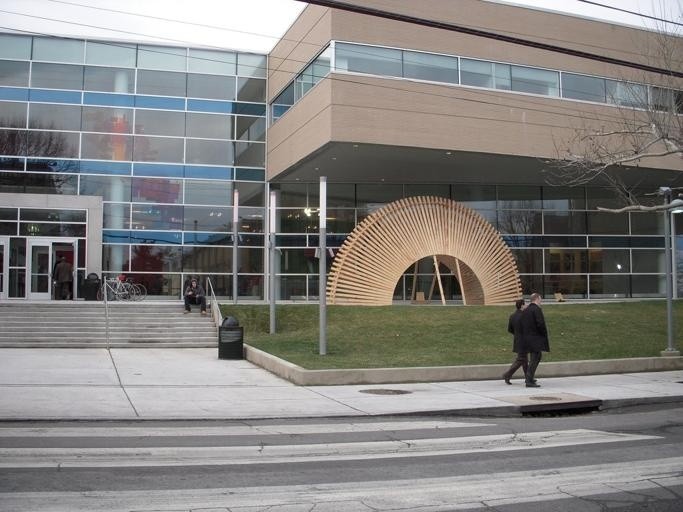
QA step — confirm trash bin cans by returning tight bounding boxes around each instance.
[85,273,100,300]
[219,316,243,360]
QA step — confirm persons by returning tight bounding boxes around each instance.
[502,299,536,385]
[183,278,207,316]
[51,254,73,300]
[520,293,550,387]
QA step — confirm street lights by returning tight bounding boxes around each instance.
[268,190,278,334]
[231,186,239,305]
[657,184,682,356]
[316,174,329,356]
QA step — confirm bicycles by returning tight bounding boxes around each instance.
[95,274,148,301]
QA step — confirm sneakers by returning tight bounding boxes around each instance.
[200,310,207,315]
[502,373,512,385]
[182,309,192,314]
[525,379,540,387]
